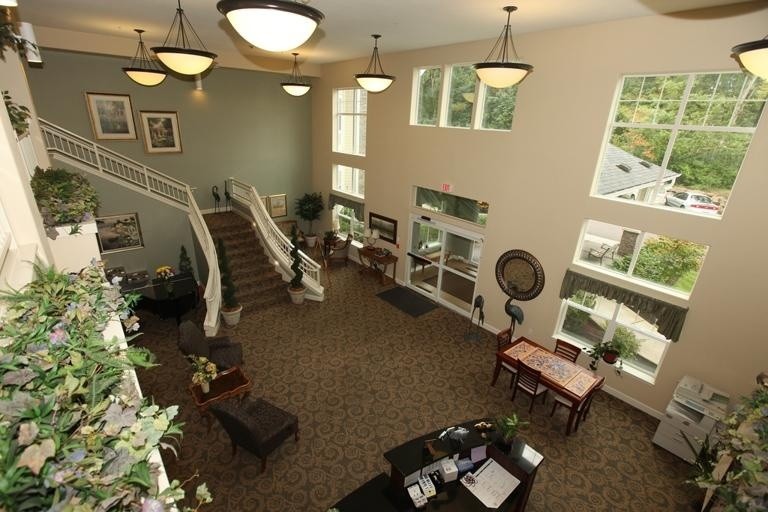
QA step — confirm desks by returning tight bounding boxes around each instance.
[323,417,538,511]
[492,336,601,436]
[121,272,199,321]
[357,246,398,286]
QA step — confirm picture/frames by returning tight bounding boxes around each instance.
[137,110,182,153]
[259,193,287,218]
[94,212,144,255]
[369,212,397,244]
[85,91,138,140]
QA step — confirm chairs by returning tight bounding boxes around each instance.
[510,358,549,414]
[551,376,606,432]
[177,321,242,371]
[587,243,618,266]
[490,329,517,389]
[327,234,353,265]
[552,338,581,363]
[209,396,298,473]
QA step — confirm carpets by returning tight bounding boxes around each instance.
[376,285,437,318]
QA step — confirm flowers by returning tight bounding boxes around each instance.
[156,265,176,279]
[184,353,217,385]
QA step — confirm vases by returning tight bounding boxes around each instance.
[201,382,210,393]
[164,280,174,293]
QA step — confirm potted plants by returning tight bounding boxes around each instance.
[218,237,242,326]
[287,223,307,305]
[495,413,529,448]
[294,190,324,248]
[582,325,641,377]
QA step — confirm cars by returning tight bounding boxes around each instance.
[666,188,721,217]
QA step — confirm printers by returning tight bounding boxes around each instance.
[653,375,731,464]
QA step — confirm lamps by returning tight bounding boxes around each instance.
[473,6,533,89]
[280,53,310,97]
[364,229,379,250]
[150,0,217,75]
[730,35,768,80]
[352,34,395,93]
[121,29,167,87]
[217,1,324,52]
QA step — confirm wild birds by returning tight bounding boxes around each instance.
[468,295,485,343]
[505,285,524,333]
